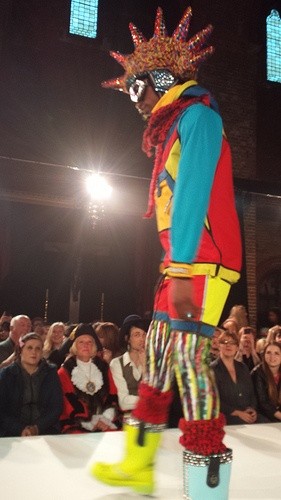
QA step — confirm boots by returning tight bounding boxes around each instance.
[91,414,166,495]
[183,448,233,500]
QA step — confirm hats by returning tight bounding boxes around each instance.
[122,314,149,335]
[60,323,103,355]
[101,7,215,95]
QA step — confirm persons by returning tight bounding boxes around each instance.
[0,333,64,436]
[111,314,151,428]
[267,324,281,344]
[212,328,228,355]
[1,315,32,364]
[222,304,249,336]
[220,319,238,334]
[95,322,124,362]
[57,323,122,433]
[236,327,257,363]
[267,307,281,326]
[32,318,45,337]
[250,342,281,423]
[89,4,242,500]
[209,332,269,425]
[43,322,68,358]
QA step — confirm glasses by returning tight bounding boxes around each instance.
[128,79,151,103]
[220,339,237,344]
